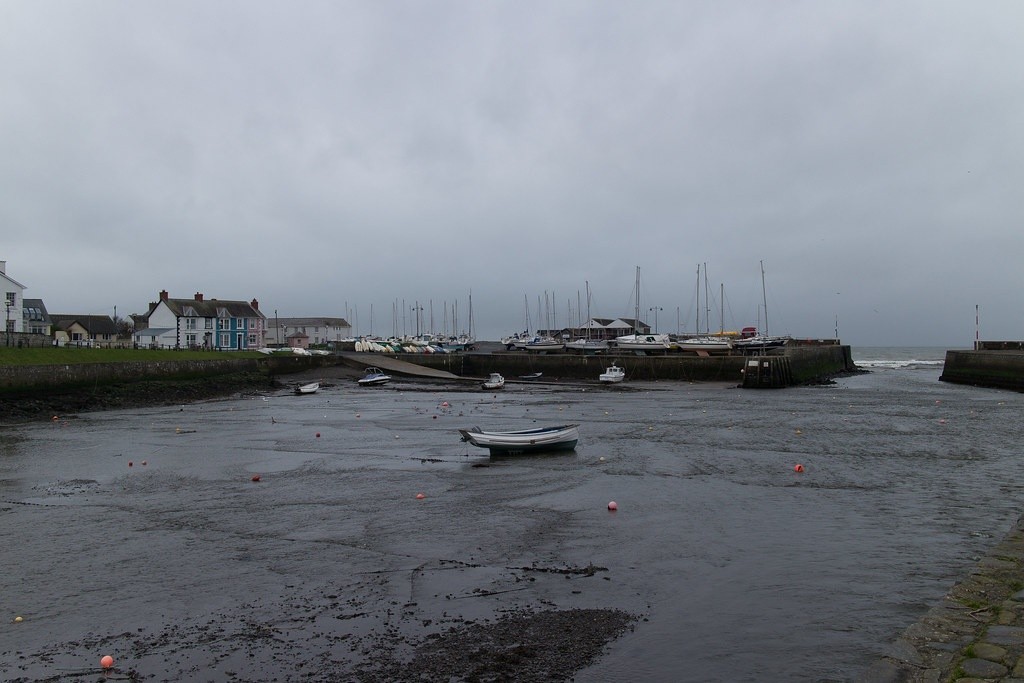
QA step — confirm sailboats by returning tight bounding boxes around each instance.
[324,286,480,353]
[500,279,619,355]
[676,258,790,354]
[615,265,671,351]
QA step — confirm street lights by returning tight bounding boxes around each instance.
[649,306,664,335]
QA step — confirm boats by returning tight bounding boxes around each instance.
[458,422,581,457]
[599,358,626,385]
[297,381,320,394]
[357,366,392,387]
[481,372,506,391]
[518,372,543,381]
